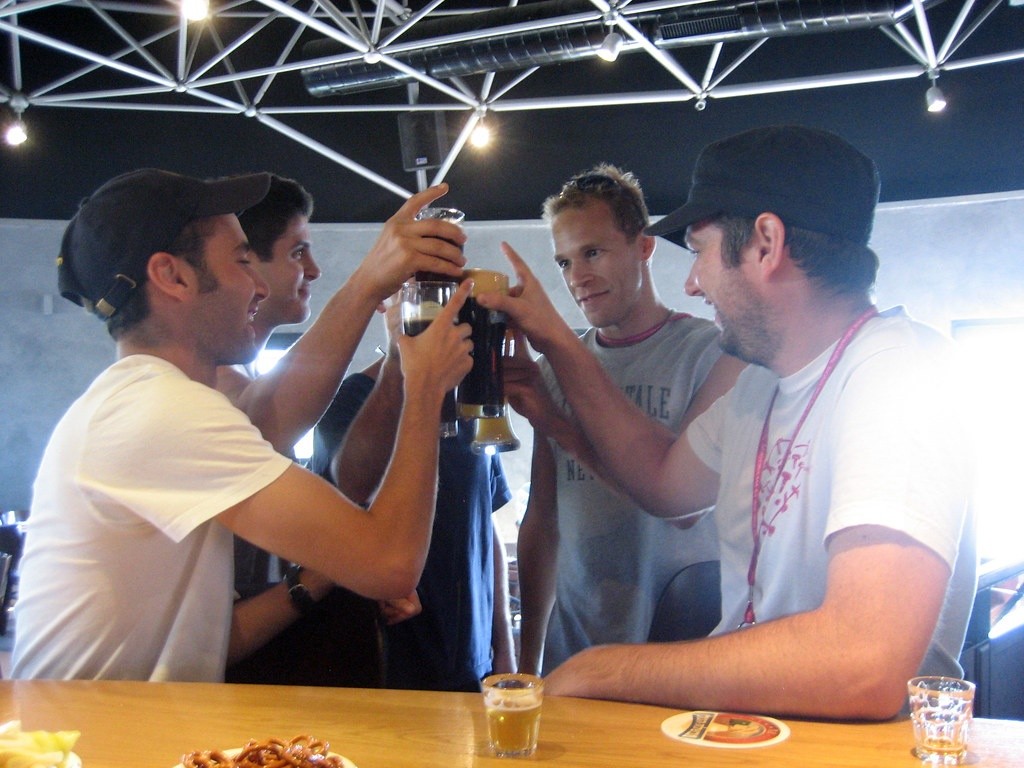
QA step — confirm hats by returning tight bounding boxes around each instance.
[643,124,880,250]
[57,167,271,321]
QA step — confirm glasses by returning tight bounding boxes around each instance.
[555,174,647,227]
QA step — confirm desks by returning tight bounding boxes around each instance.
[0,678,1024,768]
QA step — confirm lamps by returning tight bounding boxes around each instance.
[596,11,623,63]
[5,104,28,146]
[470,108,493,148]
[925,68,947,112]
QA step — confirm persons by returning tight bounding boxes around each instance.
[7,124,981,721]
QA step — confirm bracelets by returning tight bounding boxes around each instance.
[284,565,316,612]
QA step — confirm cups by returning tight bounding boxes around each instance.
[909,676,976,765]
[414,207,465,281]
[470,418,522,456]
[482,673,545,758]
[401,282,459,438]
[456,271,511,418]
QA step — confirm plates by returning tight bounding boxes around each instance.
[173,747,357,768]
